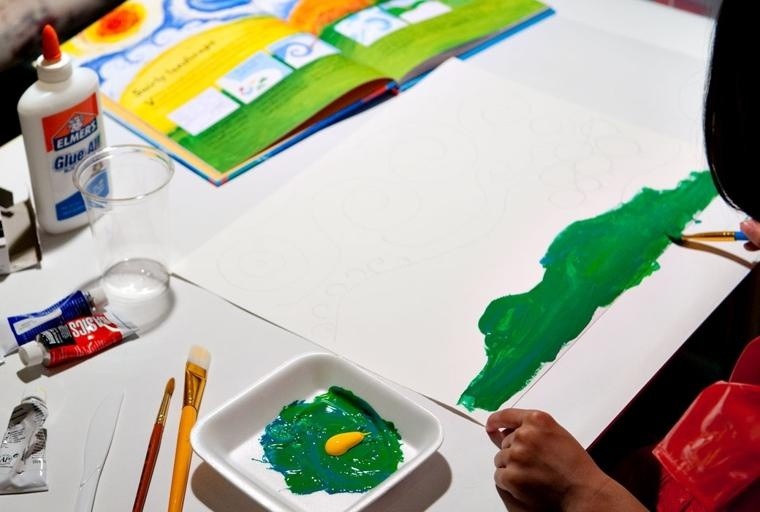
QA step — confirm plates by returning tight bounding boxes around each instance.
[188,350,445,512]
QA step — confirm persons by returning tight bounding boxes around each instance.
[484,1,760,512]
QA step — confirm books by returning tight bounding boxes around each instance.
[27,2,557,188]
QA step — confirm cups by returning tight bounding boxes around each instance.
[73,143,174,304]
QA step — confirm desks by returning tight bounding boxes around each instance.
[1,0,758,512]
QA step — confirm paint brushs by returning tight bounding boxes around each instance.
[665,231,749,241]
[168,347,210,512]
[132,378,175,512]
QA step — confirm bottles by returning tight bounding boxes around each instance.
[16,25,114,234]
